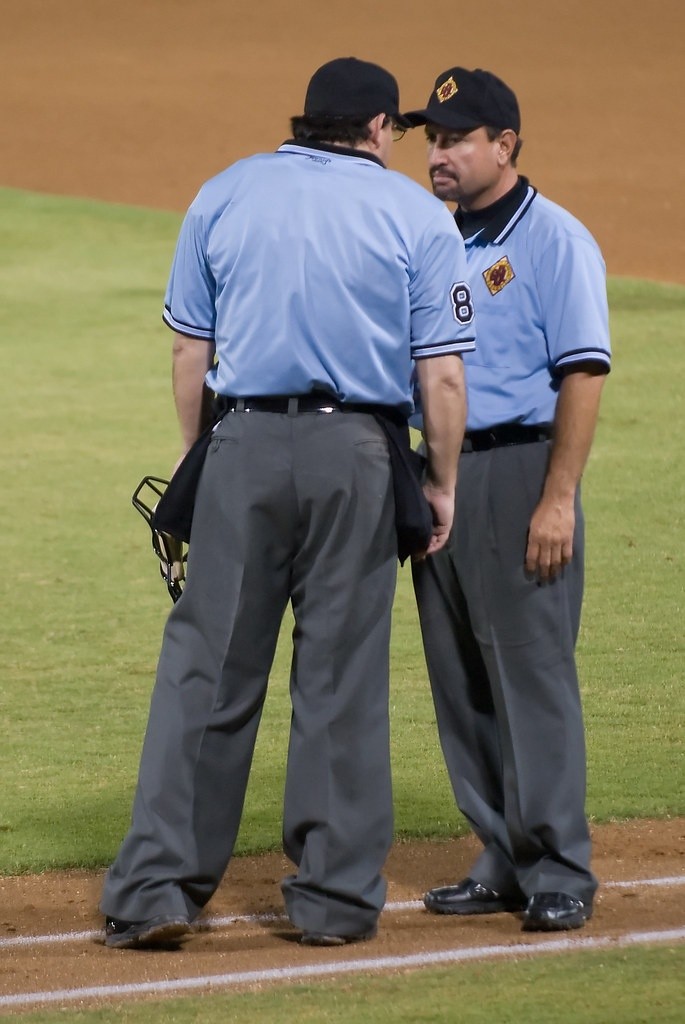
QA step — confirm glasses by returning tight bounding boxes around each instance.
[392,122,407,142]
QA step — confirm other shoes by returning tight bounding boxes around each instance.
[297,928,344,947]
[104,912,193,949]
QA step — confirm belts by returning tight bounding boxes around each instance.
[223,396,364,413]
[464,425,559,452]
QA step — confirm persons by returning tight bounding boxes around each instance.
[97,56,478,944]
[400,66,611,927]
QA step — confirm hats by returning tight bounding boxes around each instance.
[303,56,414,130]
[397,67,521,137]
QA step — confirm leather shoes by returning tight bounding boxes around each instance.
[520,892,594,932]
[423,878,522,915]
